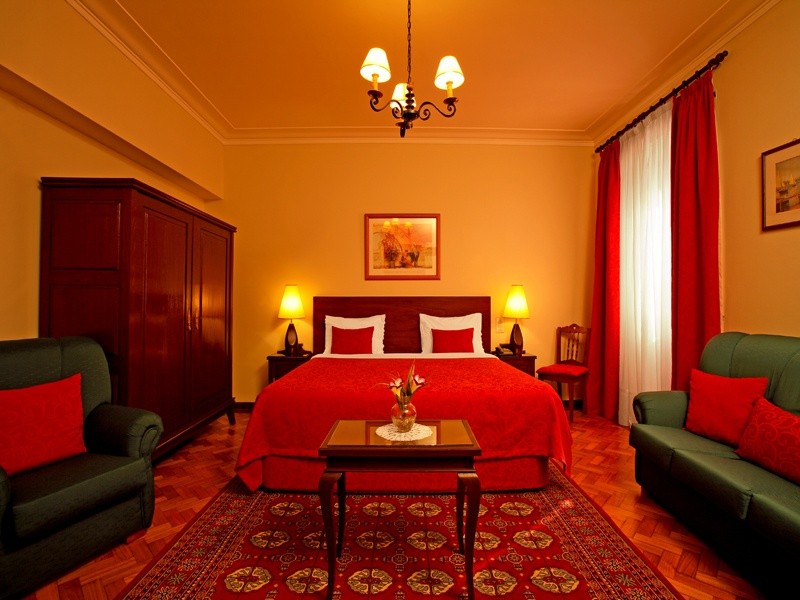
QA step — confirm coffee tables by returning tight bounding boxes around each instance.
[316,418,482,600]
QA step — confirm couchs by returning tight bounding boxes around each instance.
[627,328,800,600]
[0,333,165,600]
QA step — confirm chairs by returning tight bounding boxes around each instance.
[535,323,592,423]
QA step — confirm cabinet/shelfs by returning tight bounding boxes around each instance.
[37,174,239,470]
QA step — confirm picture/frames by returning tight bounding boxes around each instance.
[364,213,441,282]
[760,136,800,232]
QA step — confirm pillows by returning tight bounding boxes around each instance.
[418,312,486,354]
[733,393,800,486]
[681,368,770,447]
[323,314,386,355]
[0,372,88,476]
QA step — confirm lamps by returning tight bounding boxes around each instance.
[277,284,306,356]
[358,0,466,139]
[503,283,530,355]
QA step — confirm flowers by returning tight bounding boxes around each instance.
[361,359,432,420]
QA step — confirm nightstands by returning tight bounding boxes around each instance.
[266,350,313,385]
[490,349,537,378]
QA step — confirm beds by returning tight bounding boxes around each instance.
[232,294,574,495]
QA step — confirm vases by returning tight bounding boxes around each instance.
[391,400,416,434]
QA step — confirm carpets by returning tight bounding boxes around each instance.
[112,456,687,600]
